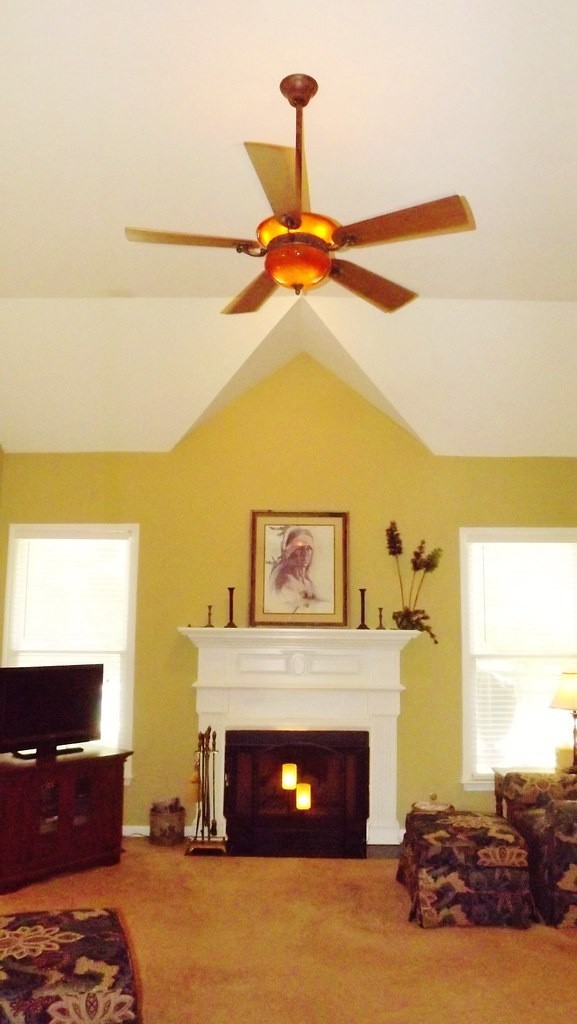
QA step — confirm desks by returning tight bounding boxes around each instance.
[492,766,577,820]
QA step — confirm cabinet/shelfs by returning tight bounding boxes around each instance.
[0,747,133,896]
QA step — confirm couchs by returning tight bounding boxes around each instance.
[503,772,577,929]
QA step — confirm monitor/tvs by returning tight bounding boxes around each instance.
[0,664,104,757]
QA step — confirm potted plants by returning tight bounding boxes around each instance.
[386,521,445,644]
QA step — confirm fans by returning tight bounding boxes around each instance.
[125,73,468,315]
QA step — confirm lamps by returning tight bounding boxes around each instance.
[549,669,577,774]
[255,212,338,295]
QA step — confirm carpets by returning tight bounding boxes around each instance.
[0,906,142,1023]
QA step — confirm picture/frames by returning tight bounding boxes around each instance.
[249,511,348,627]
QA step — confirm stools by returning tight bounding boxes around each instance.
[395,811,540,929]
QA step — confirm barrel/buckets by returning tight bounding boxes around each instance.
[149,807,185,846]
